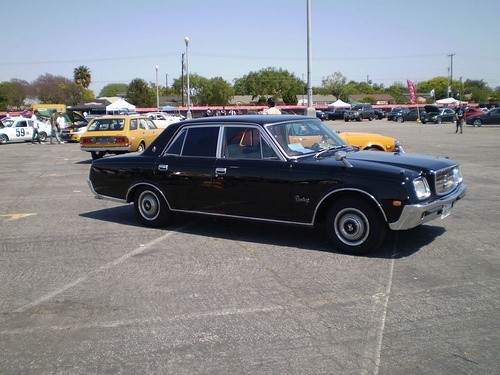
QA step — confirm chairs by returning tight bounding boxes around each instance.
[288,128,298,136]
[135,124,142,129]
[113,124,121,130]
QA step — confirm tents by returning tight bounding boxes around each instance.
[436,97,468,108]
[105,98,136,115]
[328,99,351,110]
[157,104,180,115]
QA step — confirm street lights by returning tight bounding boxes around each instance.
[155,65,160,111]
[184,37,193,119]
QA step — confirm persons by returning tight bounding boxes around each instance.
[455,104,463,133]
[50,109,63,144]
[56,111,67,130]
[31,109,45,144]
[261,98,282,115]
[203,106,243,117]
[6,110,11,117]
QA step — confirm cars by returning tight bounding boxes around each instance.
[315,103,500,128]
[59,110,186,160]
[86,114,469,255]
[0,117,53,144]
[242,126,402,152]
